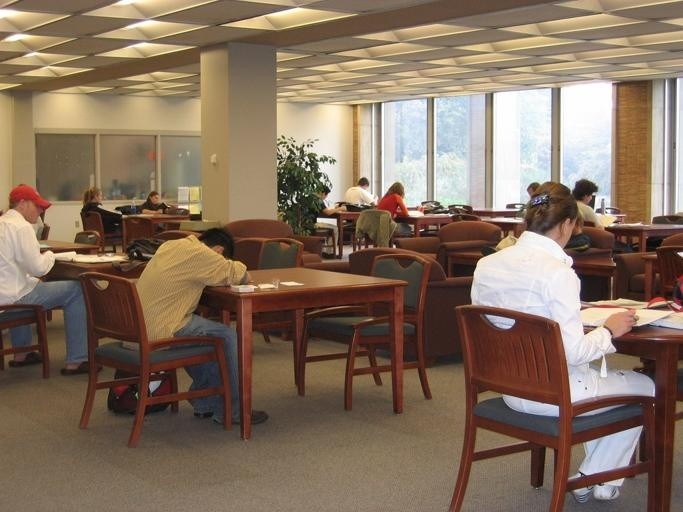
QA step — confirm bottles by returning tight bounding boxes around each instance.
[601,198,605,214]
[130,200,137,215]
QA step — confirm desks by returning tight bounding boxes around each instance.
[37,239,100,254]
[44,250,150,371]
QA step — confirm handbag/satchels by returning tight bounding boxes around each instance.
[126,237,165,259]
[107,371,172,415]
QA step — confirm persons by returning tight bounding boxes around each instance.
[527,183,540,197]
[82,187,122,233]
[564,210,591,250]
[377,182,411,236]
[121,228,269,425]
[140,191,168,232]
[0,184,103,375]
[470,182,656,504]
[302,185,346,259]
[573,179,635,252]
[347,178,375,208]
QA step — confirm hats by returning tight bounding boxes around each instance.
[9,184,51,209]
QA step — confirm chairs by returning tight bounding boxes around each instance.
[41,223,50,240]
[448,305,655,512]
[0,303,50,379]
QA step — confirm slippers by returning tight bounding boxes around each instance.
[60,362,103,376]
[9,352,41,367]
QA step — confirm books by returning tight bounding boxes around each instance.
[157,201,172,210]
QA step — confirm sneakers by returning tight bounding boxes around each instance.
[193,408,267,425]
[322,251,341,259]
[481,247,497,257]
[567,472,619,504]
